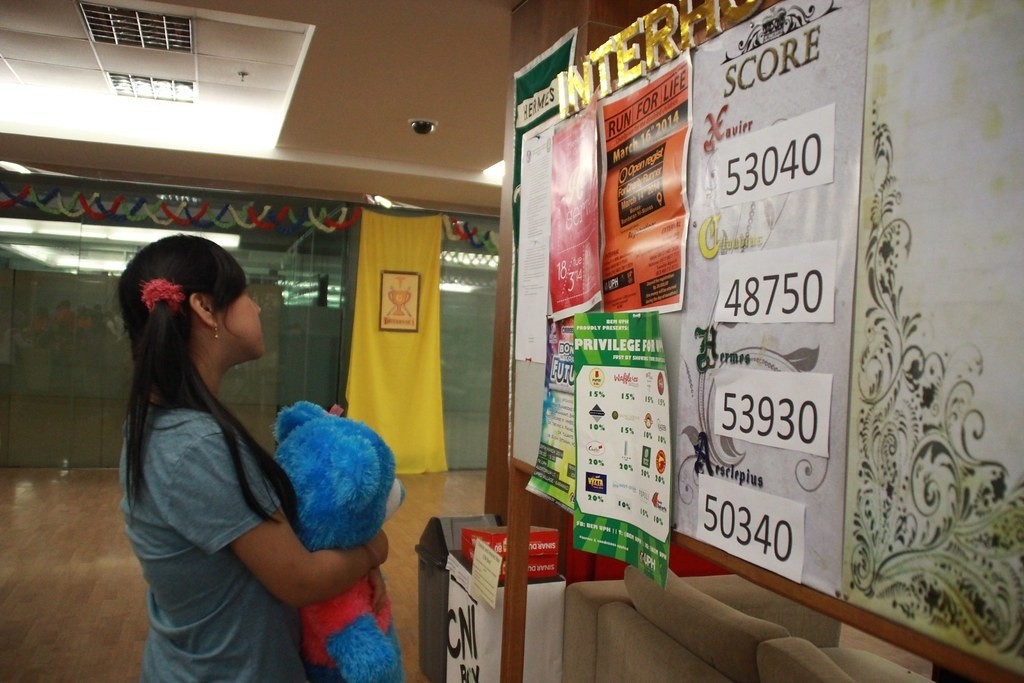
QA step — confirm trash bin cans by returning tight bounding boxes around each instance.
[416,514,500,683]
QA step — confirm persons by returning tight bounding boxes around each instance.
[116,235,391,683]
[0,295,125,399]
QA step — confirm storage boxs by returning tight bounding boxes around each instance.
[461,524,559,558]
[497,556,557,581]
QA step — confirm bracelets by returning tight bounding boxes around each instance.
[363,545,379,568]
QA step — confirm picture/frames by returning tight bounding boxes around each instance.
[379,270,421,333]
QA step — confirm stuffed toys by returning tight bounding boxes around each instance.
[273,401,406,683]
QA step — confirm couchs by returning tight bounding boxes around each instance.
[564,562,935,683]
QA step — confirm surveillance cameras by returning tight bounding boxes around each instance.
[408,120,438,134]
